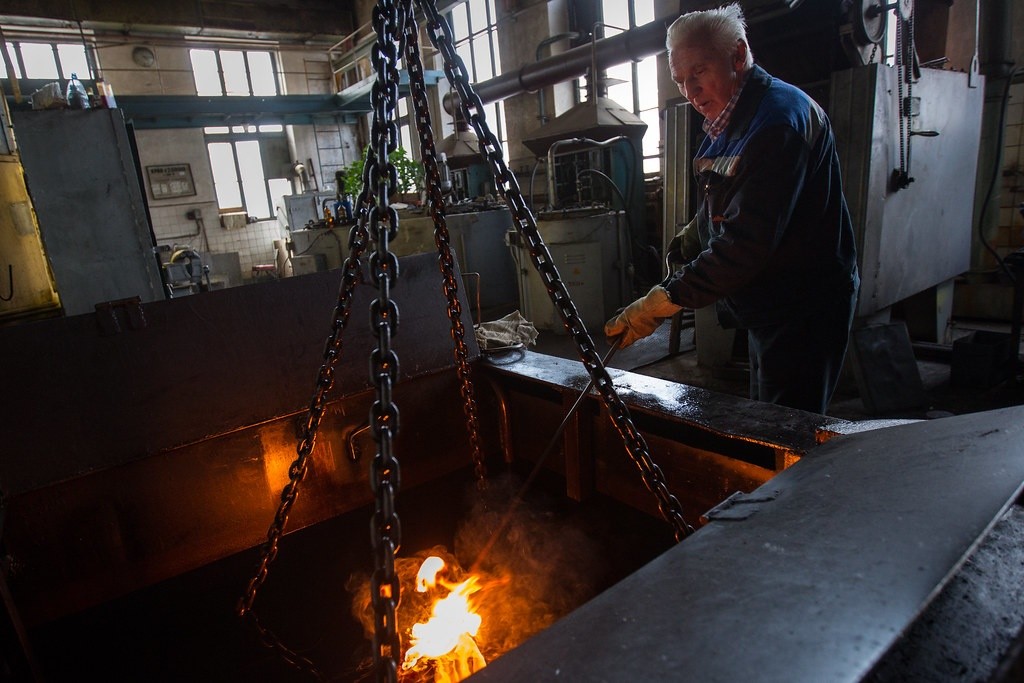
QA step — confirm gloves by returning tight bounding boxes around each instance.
[603,284,684,348]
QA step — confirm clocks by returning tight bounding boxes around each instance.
[132,46,153,67]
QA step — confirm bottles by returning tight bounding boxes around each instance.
[66,72,92,110]
[346,193,354,221]
[30,81,65,110]
[323,205,334,228]
[407,205,412,210]
[417,200,422,209]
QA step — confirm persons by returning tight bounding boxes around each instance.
[604,4,861,417]
